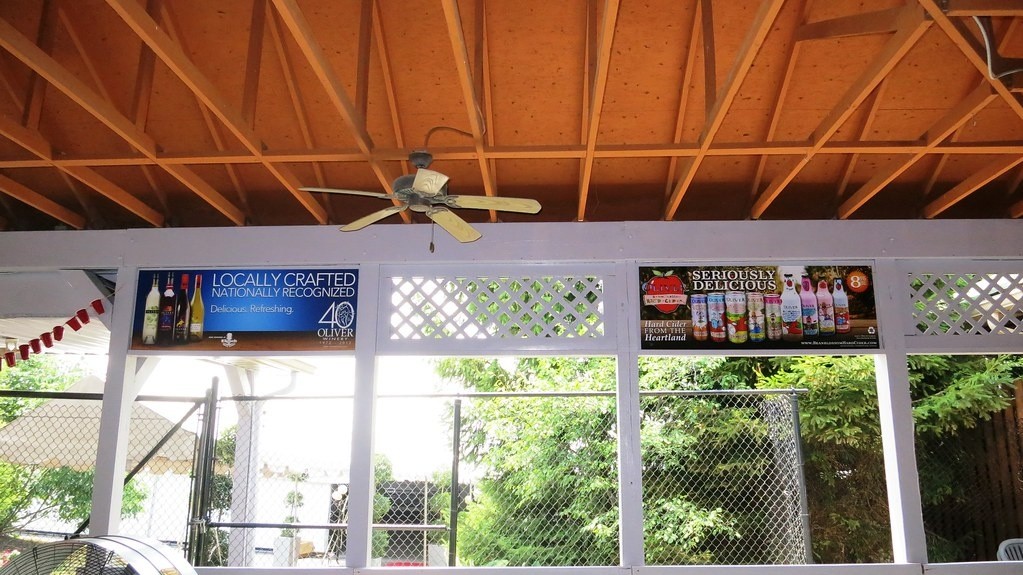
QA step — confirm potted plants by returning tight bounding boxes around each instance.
[273,469,309,566]
[428,468,459,567]
[371,453,392,566]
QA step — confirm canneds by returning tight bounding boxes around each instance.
[691,291,782,344]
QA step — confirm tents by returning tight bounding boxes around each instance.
[0,374,201,475]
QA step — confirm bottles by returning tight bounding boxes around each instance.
[780,273,803,339]
[173,275,191,343]
[815,275,836,336]
[800,273,820,337]
[142,274,161,345]
[190,275,205,341]
[832,277,850,332]
[158,273,175,346]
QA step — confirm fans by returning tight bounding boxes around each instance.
[297,148,541,254]
